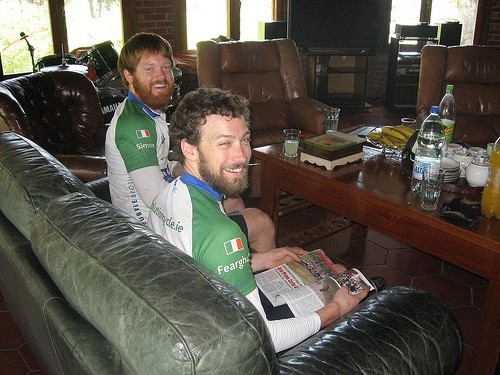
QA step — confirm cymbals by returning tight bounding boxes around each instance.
[3,30,37,51]
[41,65,88,74]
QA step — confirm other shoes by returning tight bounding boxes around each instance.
[359,276,387,303]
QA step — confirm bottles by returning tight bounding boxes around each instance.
[438,84,457,143]
[482,136,500,220]
[410,106,447,197]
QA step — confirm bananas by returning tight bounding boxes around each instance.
[367,125,417,148]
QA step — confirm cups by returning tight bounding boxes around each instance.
[420,166,445,211]
[400,118,417,130]
[323,107,340,133]
[442,143,495,188]
[283,129,302,158]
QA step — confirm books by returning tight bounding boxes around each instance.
[253,248,375,317]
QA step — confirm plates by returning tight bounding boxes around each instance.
[414,156,461,182]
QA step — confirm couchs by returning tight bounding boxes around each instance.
[196,38,328,199]
[0,70,112,183]
[416,45,500,150]
[0,130,463,375]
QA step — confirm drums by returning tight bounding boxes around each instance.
[76,40,121,85]
[35,53,77,71]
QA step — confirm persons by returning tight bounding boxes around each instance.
[147,85,387,353]
[105,32,276,253]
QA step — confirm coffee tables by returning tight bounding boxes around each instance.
[252,144,500,375]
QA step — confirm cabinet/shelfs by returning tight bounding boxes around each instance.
[301,50,376,113]
[384,37,438,113]
